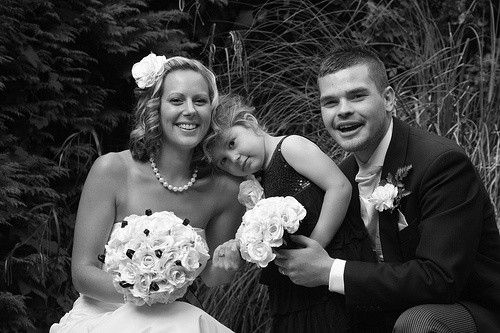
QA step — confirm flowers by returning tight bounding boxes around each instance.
[130,52,169,91]
[233,193,306,270]
[238,178,267,210]
[98,207,212,309]
[367,164,411,215]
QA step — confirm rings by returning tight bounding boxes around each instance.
[282,269,287,276]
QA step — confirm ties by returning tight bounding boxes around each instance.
[357,164,382,246]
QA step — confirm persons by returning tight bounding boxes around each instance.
[275,44,500,333]
[203,93,378,332]
[54,54,277,333]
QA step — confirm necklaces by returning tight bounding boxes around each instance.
[148,153,202,193]
